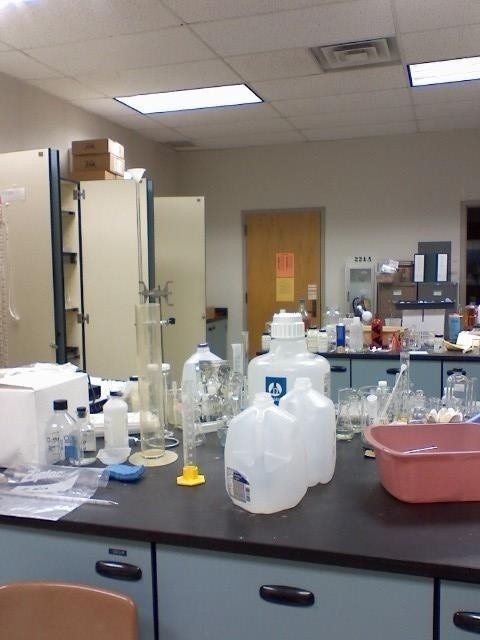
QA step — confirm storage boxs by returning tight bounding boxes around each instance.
[1,370,90,471]
[363,325,404,347]
[70,138,126,181]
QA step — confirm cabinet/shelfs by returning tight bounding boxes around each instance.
[325,359,480,421]
[0,523,478,640]
[207,318,228,359]
[2,148,207,381]
[458,201,480,308]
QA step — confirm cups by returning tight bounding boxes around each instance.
[199,360,247,448]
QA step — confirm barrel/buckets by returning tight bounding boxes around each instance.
[181,308,364,514]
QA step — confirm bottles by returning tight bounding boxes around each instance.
[401,322,425,351]
[48,391,129,469]
[449,313,461,343]
[127,375,142,415]
[337,337,480,439]
[296,299,385,354]
[178,383,205,488]
[134,303,168,460]
[465,305,476,332]
